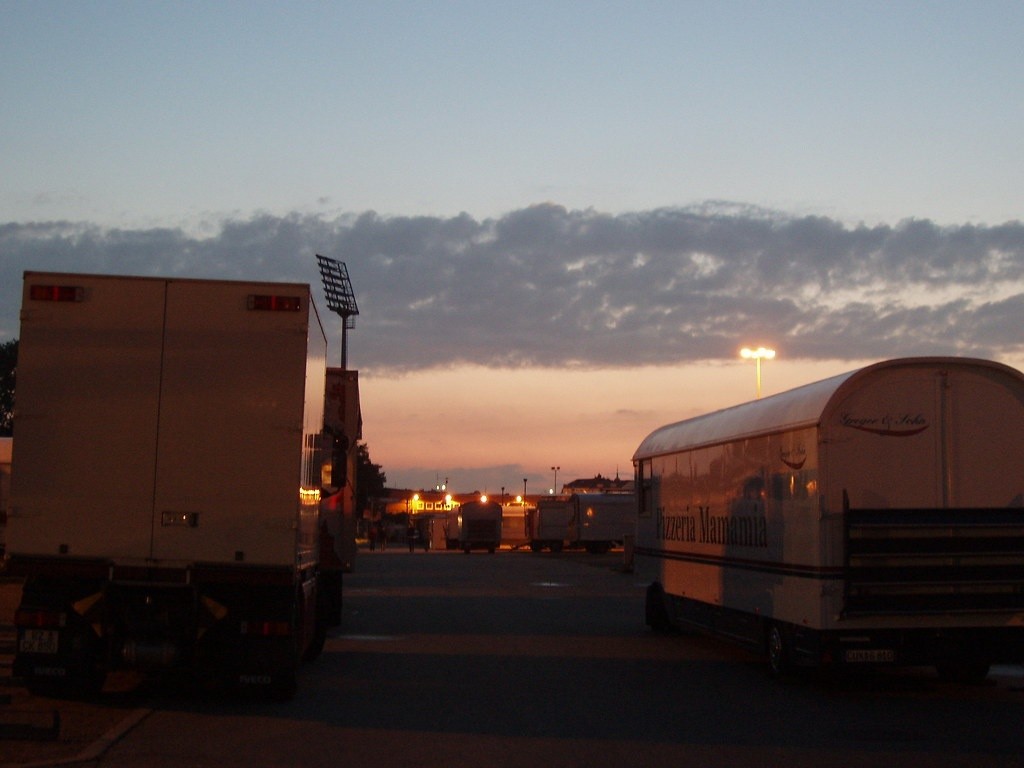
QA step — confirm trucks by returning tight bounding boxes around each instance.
[630,355,1024,680]
[524,491,632,556]
[0,267,351,699]
[461,499,502,554]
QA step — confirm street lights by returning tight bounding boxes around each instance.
[741,348,778,400]
[551,466,560,494]
[501,486,504,506]
[523,478,528,505]
